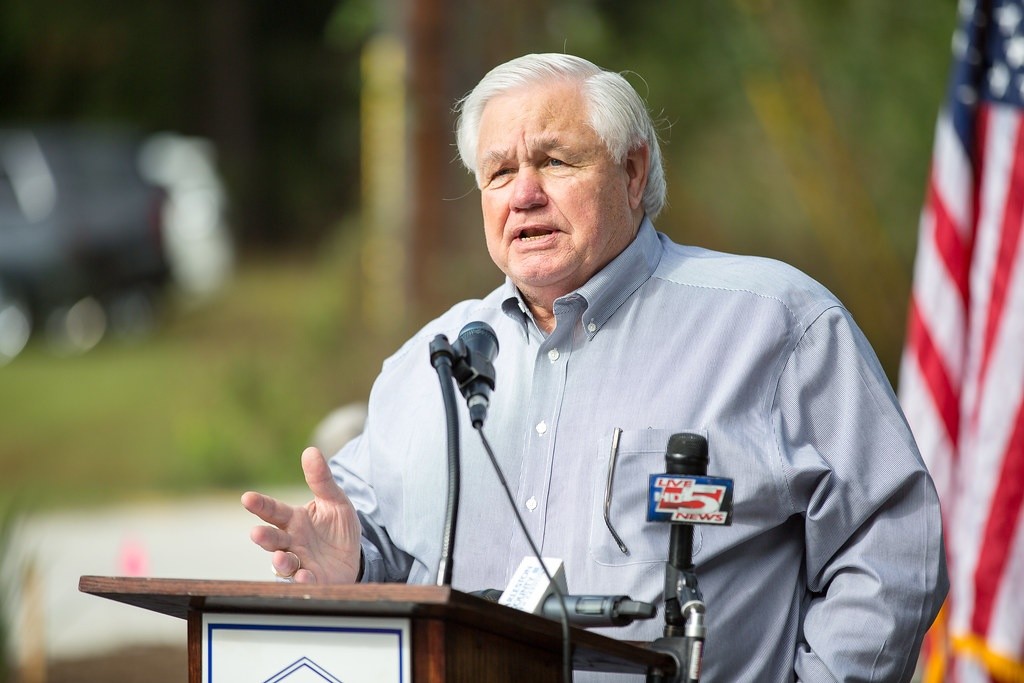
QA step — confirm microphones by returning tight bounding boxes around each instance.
[458,321,499,429]
[467,555,657,627]
[646,433,734,637]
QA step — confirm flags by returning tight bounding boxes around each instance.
[897,1,1024,683]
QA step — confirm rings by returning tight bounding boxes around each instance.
[273,552,300,578]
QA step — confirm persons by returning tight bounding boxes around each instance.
[242,51,950,683]
